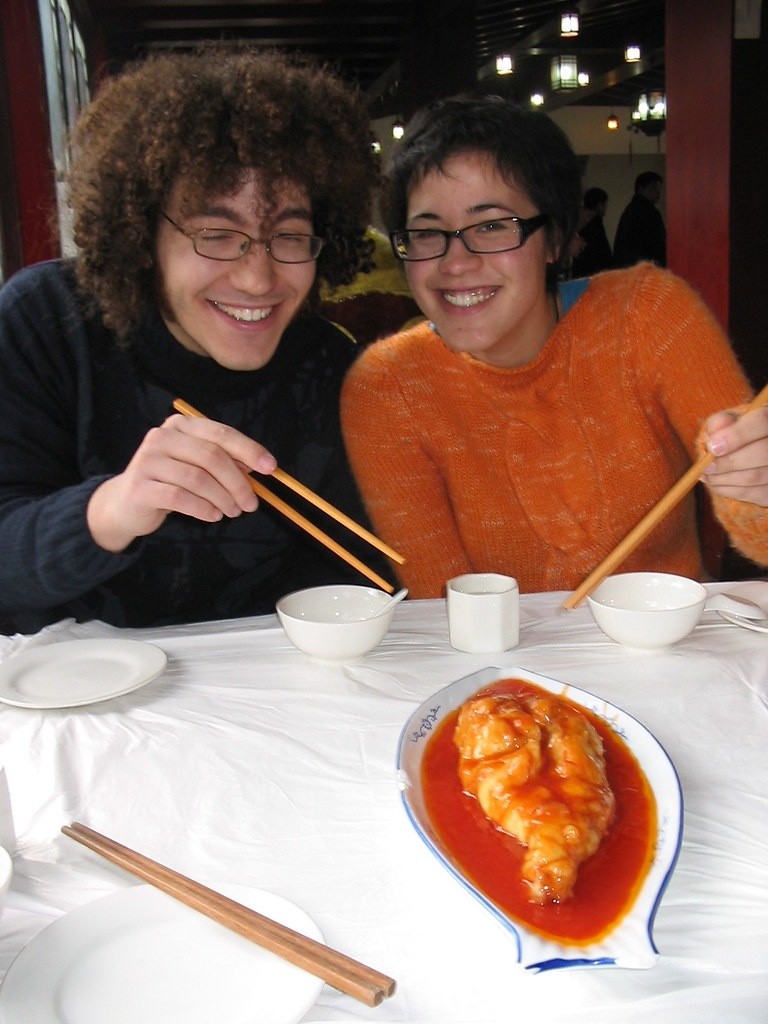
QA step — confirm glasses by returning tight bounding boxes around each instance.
[156,205,328,264]
[391,213,549,261]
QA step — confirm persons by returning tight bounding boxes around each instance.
[1,49,376,637]
[570,188,612,281]
[339,96,767,602]
[612,171,665,269]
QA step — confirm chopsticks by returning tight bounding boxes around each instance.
[61,822,396,1006]
[165,399,405,592]
[564,385,768,609]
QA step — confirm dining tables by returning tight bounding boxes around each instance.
[0,583,768,1024]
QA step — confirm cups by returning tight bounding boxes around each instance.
[446,574,520,654]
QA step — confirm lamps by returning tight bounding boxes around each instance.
[391,114,404,138]
[495,4,669,139]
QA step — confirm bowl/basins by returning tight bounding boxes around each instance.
[276,585,394,659]
[586,572,707,648]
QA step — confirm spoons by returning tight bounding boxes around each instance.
[704,593,768,619]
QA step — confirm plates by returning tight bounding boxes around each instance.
[0,639,167,709]
[0,885,326,1024]
[718,582,768,634]
[396,668,686,977]
